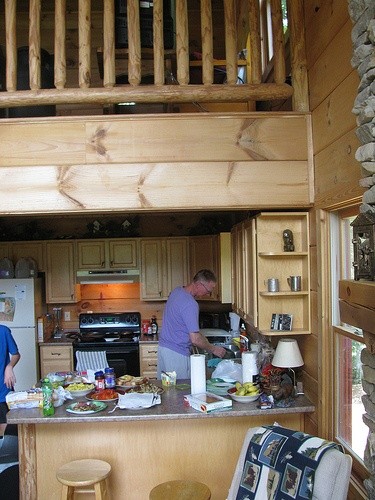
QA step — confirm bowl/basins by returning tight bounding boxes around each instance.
[226,387,264,403]
[65,386,95,397]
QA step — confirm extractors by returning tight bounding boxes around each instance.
[75,268,140,284]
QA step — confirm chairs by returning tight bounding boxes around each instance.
[75,350,110,373]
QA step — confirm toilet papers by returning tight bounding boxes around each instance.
[242,351,258,384]
[189,354,206,396]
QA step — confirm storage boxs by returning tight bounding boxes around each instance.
[183,390,232,414]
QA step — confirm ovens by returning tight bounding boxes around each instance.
[72,346,140,379]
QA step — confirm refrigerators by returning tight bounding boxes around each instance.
[0,275,43,392]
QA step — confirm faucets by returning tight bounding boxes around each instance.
[238,334,250,343]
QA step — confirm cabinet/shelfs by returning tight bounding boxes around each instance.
[138,341,158,378]
[0,212,313,337]
[40,345,74,380]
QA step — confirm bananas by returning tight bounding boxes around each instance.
[235,381,260,396]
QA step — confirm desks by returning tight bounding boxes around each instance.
[5,383,317,500]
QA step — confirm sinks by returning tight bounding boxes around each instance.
[201,352,244,359]
[197,345,240,353]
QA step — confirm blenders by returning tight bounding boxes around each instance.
[53,307,65,339]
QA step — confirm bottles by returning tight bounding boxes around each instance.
[95,366,114,390]
[267,279,279,292]
[0,256,38,278]
[143,315,158,336]
[42,378,55,416]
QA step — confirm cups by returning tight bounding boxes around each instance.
[287,276,301,292]
[161,372,176,387]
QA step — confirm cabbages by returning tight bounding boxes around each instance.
[65,382,94,390]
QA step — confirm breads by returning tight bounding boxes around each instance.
[115,374,147,386]
[6,390,58,409]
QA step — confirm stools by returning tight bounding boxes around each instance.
[149,480,212,500]
[56,458,111,500]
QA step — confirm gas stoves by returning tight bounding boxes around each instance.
[72,312,141,345]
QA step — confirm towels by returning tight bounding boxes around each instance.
[75,350,109,372]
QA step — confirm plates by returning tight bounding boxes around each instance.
[116,384,138,388]
[85,390,121,402]
[126,385,165,394]
[65,401,107,414]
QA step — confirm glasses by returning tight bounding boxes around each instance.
[199,282,211,294]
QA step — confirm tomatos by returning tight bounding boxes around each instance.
[92,388,118,401]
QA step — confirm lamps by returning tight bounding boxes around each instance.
[271,339,304,395]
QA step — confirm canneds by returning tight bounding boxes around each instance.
[94,368,116,391]
[142,320,152,335]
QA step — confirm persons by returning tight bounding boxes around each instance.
[157,270,226,380]
[0,324,21,438]
